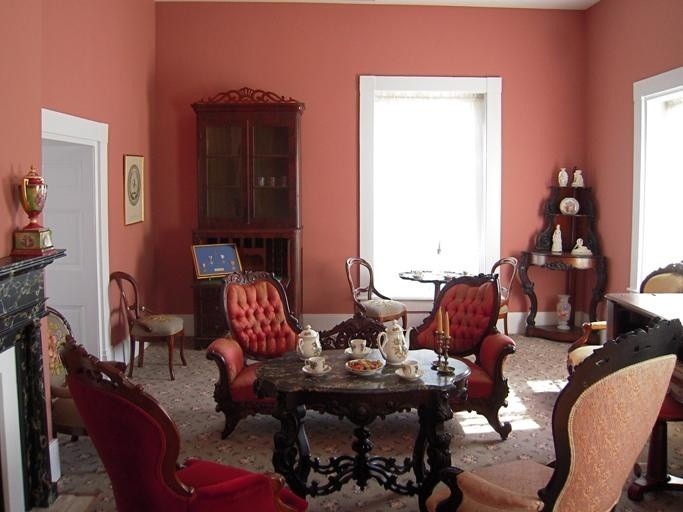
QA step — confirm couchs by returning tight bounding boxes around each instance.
[205,270,516,440]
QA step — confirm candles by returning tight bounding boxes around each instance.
[444,311,450,336]
[436,307,443,333]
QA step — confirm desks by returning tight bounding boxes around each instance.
[603,293,683,502]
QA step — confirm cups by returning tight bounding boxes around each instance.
[269,176,278,187]
[403,360,421,376]
[304,356,326,371]
[255,176,266,185]
[349,338,367,354]
[279,175,288,187]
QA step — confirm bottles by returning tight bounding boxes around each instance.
[556,168,570,187]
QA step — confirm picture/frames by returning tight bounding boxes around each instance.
[190,243,243,280]
[123,153,144,226]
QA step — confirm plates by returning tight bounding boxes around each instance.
[343,347,372,359]
[344,359,383,376]
[414,275,425,278]
[559,197,582,214]
[396,368,426,382]
[301,363,332,377]
[444,274,461,280]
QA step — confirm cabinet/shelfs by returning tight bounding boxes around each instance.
[191,86,306,349]
[519,166,608,345]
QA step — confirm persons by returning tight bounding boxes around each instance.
[551,224,563,252]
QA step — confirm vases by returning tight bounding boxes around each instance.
[558,168,569,187]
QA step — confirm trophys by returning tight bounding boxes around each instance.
[12,163,57,257]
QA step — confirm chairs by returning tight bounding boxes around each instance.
[345,258,408,334]
[47,306,127,442]
[114,271,187,381]
[567,260,683,374]
[490,257,518,335]
[59,335,309,512]
[418,319,683,512]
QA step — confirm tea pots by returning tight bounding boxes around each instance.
[376,318,414,364]
[295,324,321,361]
